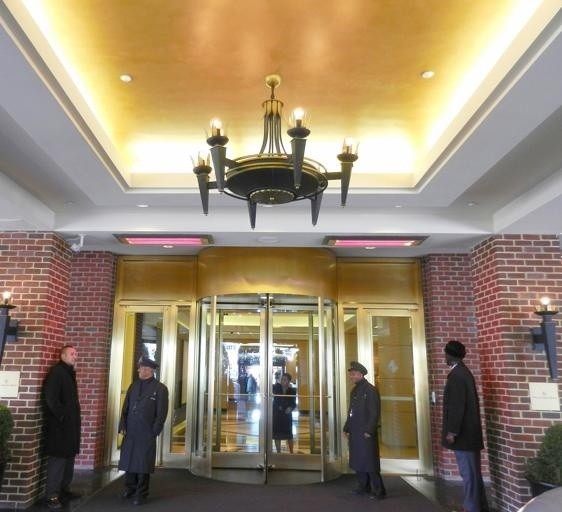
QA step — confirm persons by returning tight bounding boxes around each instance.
[441,341,490,512]
[117,356,169,506]
[272,373,296,453]
[44,346,81,512]
[342,361,387,501]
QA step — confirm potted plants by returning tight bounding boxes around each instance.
[522,423,561,497]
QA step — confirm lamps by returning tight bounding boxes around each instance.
[187,74,360,232]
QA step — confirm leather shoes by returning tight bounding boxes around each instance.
[118,486,146,505]
[46,491,81,511]
[348,488,384,501]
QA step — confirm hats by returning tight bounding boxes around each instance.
[347,360,368,375]
[136,356,156,369]
[445,341,465,358]
